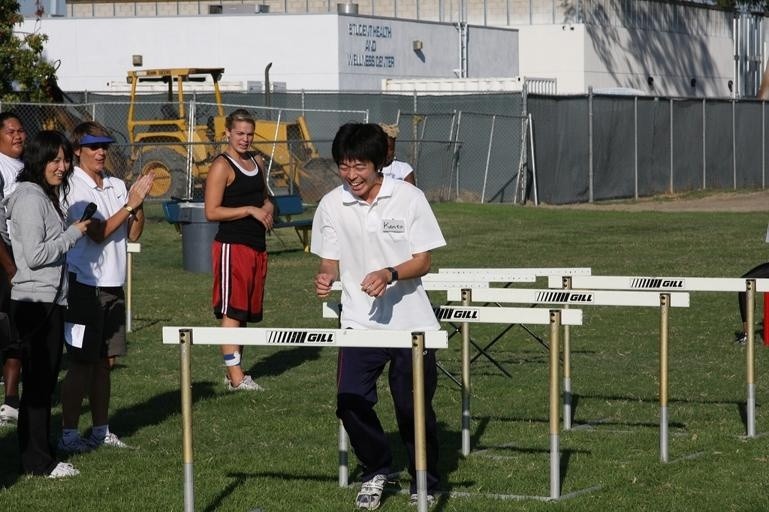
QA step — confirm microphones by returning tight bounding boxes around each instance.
[80,202,97,223]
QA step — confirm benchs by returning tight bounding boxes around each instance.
[163,196,312,252]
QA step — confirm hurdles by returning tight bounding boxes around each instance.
[336,304,601,502]
[162,325,449,511]
[548,275,769,439]
[447,288,705,471]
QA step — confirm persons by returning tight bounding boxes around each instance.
[204,108,276,395]
[307,121,448,512]
[733,223,769,344]
[376,122,417,187]
[56,120,152,454]
[0,112,33,423]
[4,129,90,481]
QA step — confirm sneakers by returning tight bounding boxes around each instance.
[225,374,263,392]
[409,494,434,506]
[48,462,80,478]
[355,474,386,510]
[0,404,19,426]
[57,433,125,450]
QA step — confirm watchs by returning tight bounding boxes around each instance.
[388,266,401,285]
[125,204,135,219]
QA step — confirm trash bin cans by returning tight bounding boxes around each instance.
[178,203,220,275]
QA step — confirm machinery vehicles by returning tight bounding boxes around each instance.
[120,64,358,210]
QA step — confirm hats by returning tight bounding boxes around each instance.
[80,135,114,144]
